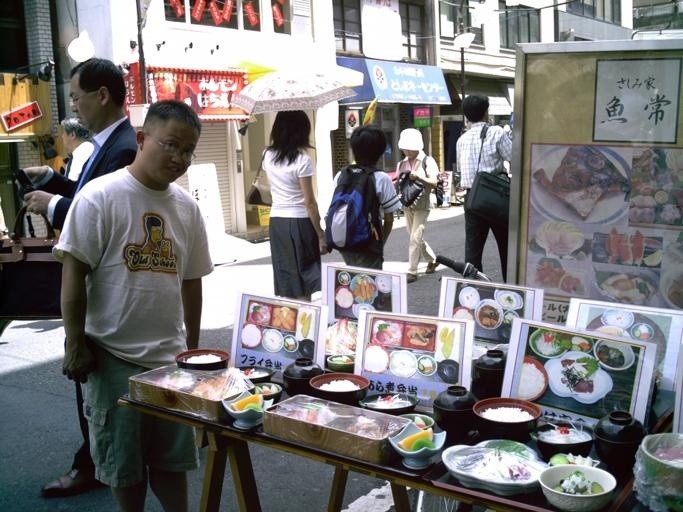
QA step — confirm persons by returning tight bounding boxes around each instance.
[454,94,512,283]
[395,128,440,283]
[331,123,402,271]
[13,59,138,497]
[0,201,10,241]
[261,110,331,303]
[59,117,94,182]
[51,99,215,512]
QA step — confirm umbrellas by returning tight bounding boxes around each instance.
[230,69,358,114]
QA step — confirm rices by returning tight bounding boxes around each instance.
[453,308,472,319]
[335,288,353,308]
[318,378,360,392]
[479,407,534,422]
[518,360,544,400]
[242,324,261,346]
[183,354,221,364]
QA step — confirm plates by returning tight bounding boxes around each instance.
[416,354,437,378]
[282,335,299,353]
[261,329,284,352]
[333,273,390,320]
[453,287,523,327]
[518,322,635,411]
[389,349,417,378]
[524,142,680,308]
[587,307,659,347]
[240,322,263,348]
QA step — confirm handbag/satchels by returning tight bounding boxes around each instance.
[392,171,425,208]
[464,171,509,229]
[244,181,272,206]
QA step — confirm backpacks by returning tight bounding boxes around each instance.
[324,164,384,257]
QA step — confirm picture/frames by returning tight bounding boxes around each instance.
[499,318,658,429]
[438,275,536,344]
[502,38,683,286]
[353,310,476,411]
[567,298,683,394]
[324,264,407,327]
[229,293,323,371]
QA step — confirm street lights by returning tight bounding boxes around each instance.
[453,15,475,134]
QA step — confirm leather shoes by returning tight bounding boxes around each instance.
[41,468,106,497]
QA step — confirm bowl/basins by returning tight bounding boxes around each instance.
[249,379,285,409]
[174,349,231,370]
[630,431,682,511]
[231,365,276,384]
[436,359,458,384]
[537,464,617,511]
[325,354,355,370]
[359,394,419,415]
[308,371,371,405]
[471,397,543,442]
[296,339,313,359]
[527,421,594,460]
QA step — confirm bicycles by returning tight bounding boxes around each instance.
[452,171,466,203]
[434,255,494,288]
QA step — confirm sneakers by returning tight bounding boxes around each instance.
[406,272,417,283]
[425,261,439,274]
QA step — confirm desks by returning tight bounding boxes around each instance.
[118,368,683,512]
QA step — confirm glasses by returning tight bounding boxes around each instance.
[142,134,198,163]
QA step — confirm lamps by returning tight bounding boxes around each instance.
[237,121,249,138]
[11,57,53,83]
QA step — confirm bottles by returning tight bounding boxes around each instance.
[146,72,177,103]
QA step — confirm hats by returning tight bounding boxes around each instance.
[398,128,424,151]
